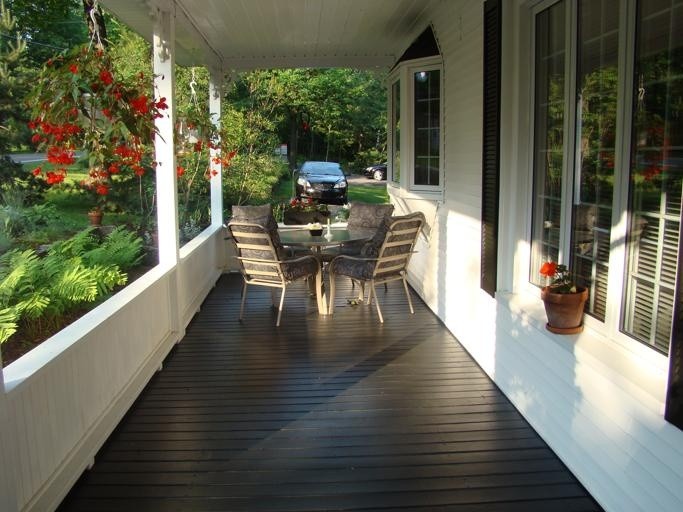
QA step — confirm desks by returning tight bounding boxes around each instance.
[278,226,377,315]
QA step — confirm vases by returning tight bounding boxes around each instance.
[284,210,331,225]
[80,93,123,129]
[188,127,199,144]
[541,285,589,334]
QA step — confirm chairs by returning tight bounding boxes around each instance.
[325,212,425,323]
[231,204,273,297]
[226,215,323,327]
[346,202,395,291]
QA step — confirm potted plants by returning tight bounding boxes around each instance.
[88,207,104,225]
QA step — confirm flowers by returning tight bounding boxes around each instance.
[28,41,168,196]
[176,107,235,180]
[286,197,325,212]
[539,262,577,294]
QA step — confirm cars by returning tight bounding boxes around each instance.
[293,159,349,206]
[273,143,287,157]
[360,161,387,181]
